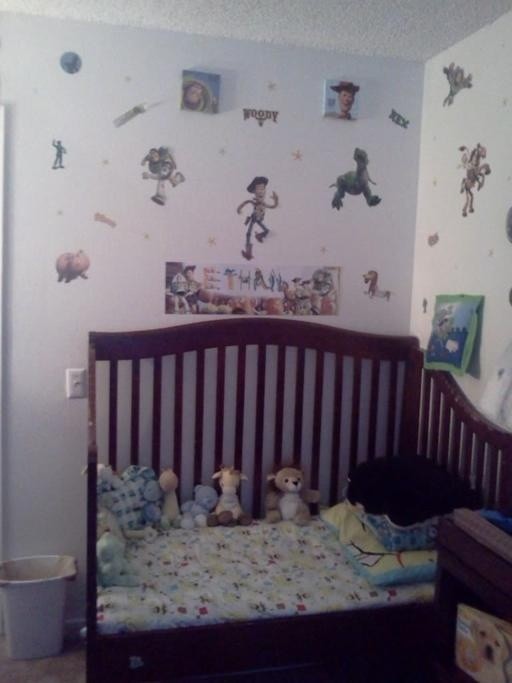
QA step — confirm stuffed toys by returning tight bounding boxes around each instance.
[96,504,160,545]
[263,465,323,525]
[97,529,144,590]
[180,482,220,530]
[213,463,255,525]
[136,476,164,528]
[156,466,184,530]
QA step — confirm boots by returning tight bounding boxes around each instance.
[242,244,253,260]
[255,232,266,243]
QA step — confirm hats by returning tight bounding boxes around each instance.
[330,80,359,93]
[184,265,196,273]
[247,176,268,193]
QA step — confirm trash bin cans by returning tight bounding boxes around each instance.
[0,555,78,660]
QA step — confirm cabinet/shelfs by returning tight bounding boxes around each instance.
[86,318,512,683]
[431,517,512,683]
